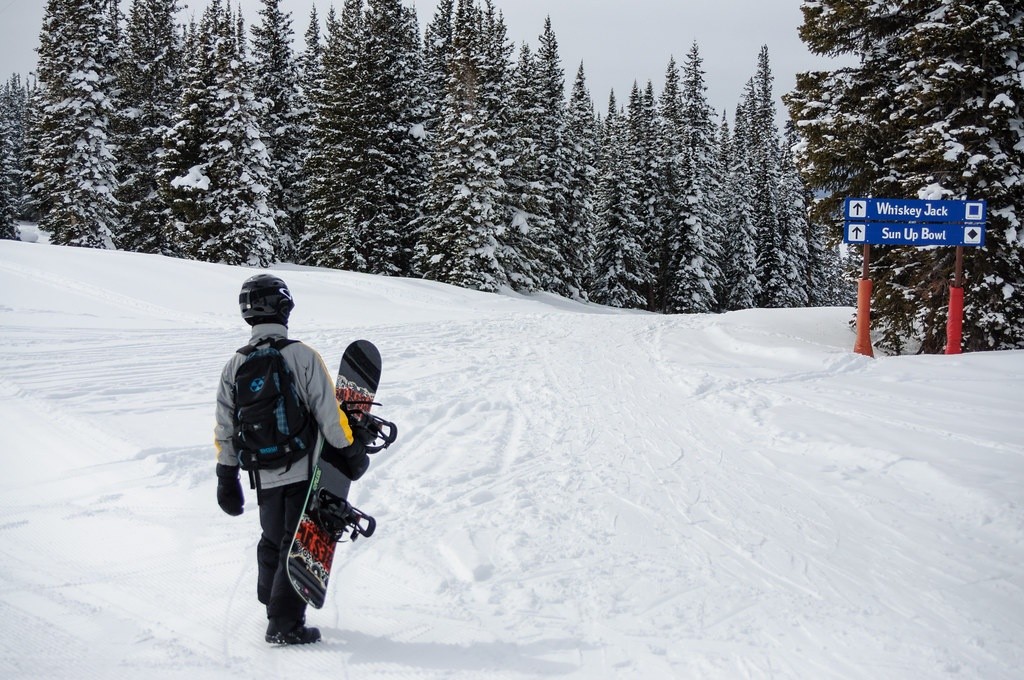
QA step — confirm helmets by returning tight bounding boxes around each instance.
[239,274,295,326]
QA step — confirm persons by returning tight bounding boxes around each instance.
[213,274,370,646]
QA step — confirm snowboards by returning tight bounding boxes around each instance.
[286,338,399,609]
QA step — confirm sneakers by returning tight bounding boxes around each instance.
[265,616,321,644]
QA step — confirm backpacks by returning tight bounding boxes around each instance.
[232,339,319,471]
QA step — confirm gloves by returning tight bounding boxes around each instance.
[216,463,244,516]
[339,438,370,481]
